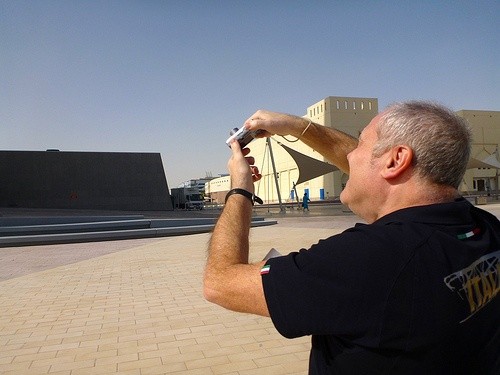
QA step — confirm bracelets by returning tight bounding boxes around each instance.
[224,188,263,206]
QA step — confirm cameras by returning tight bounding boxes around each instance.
[227,119,260,149]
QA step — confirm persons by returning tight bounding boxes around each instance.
[201,101,500,374]
[302,193,310,211]
